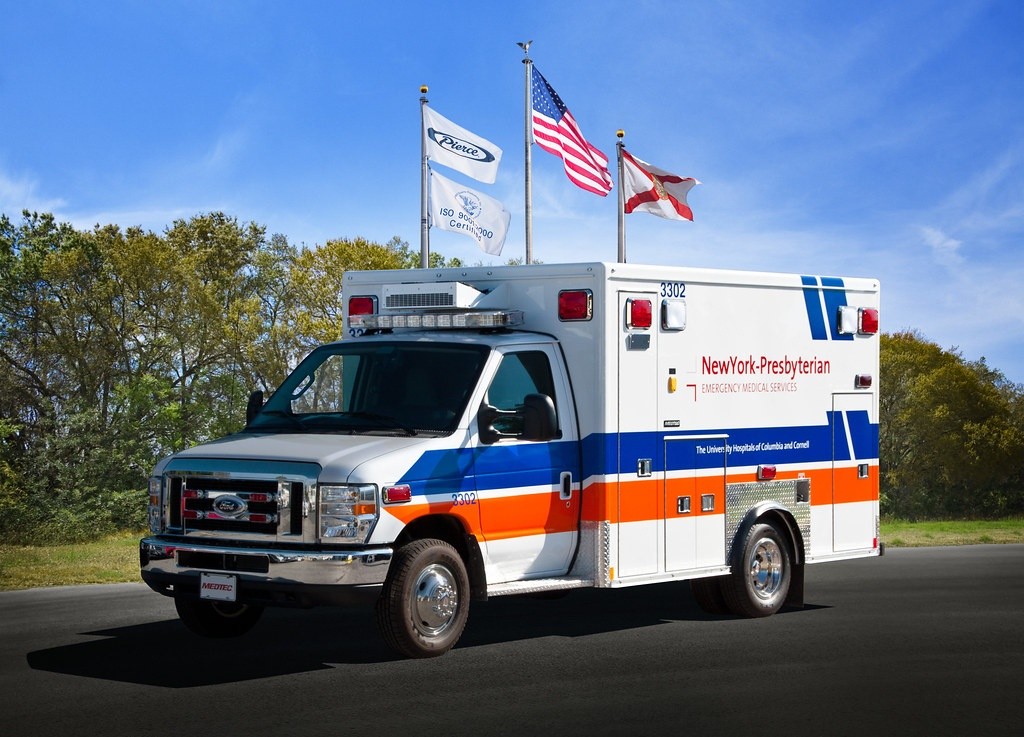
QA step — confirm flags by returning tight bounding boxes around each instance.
[428,168,511,257]
[421,104,504,184]
[622,148,702,223]
[532,63,615,197]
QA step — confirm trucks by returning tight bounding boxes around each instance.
[137,258,883,658]
